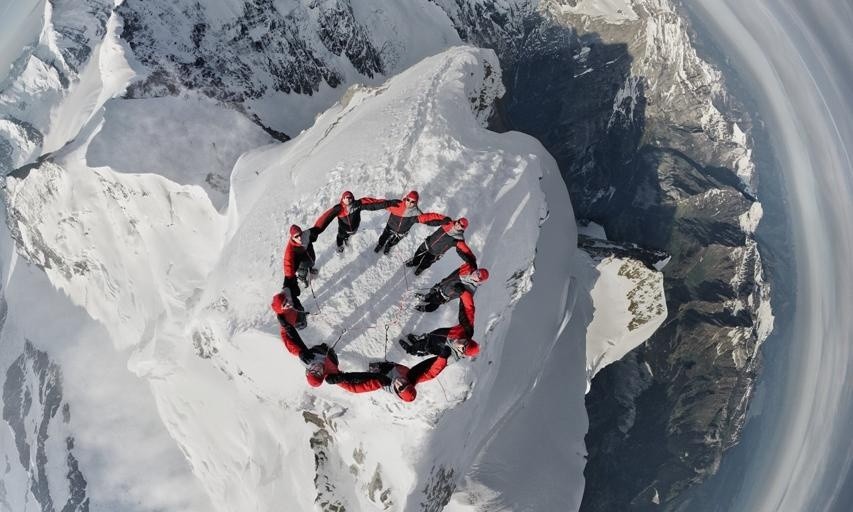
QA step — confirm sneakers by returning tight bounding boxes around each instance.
[415,293,433,312]
[374,244,392,255]
[399,334,418,355]
[406,261,424,275]
[337,237,349,252]
[303,268,319,287]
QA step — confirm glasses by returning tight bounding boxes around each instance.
[399,383,409,391]
[310,370,322,377]
[407,198,415,203]
[295,234,302,238]
[476,270,481,279]
[283,299,287,307]
[458,220,464,228]
[462,340,468,352]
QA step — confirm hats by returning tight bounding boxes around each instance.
[479,269,489,281]
[273,294,285,306]
[399,384,416,402]
[343,191,353,197]
[460,218,468,229]
[306,373,323,387]
[464,339,479,355]
[290,225,301,236]
[407,191,419,202]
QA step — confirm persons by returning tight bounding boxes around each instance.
[271,189,488,402]
